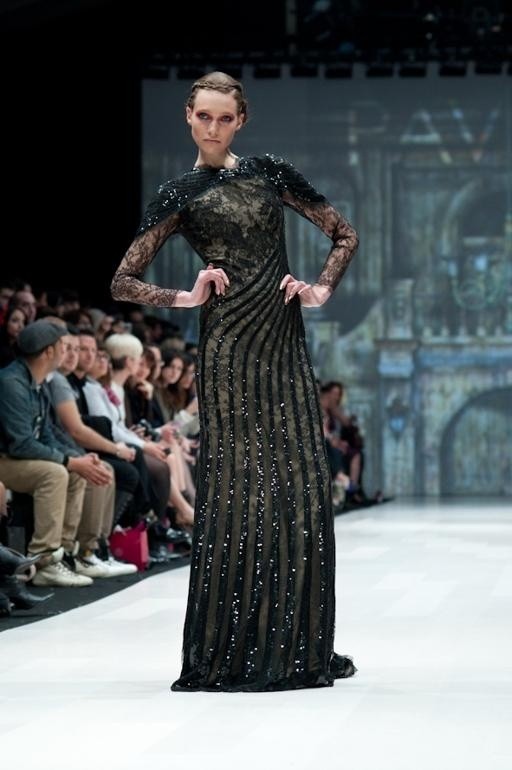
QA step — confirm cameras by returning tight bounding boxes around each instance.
[134,419,161,442]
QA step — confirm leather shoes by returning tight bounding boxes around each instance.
[150,523,191,567]
[0,541,55,612]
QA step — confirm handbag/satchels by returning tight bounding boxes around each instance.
[108,525,150,574]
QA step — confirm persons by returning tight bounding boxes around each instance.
[109,70,359,694]
[313,379,364,516]
[1,283,202,620]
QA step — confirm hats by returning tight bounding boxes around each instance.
[16,320,68,353]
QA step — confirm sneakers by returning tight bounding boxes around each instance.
[32,552,137,587]
